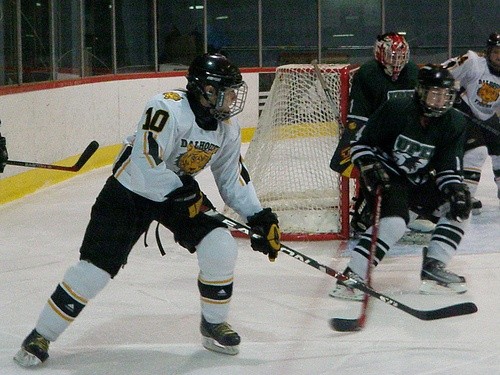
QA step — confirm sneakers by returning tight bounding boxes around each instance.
[419,247,467,295]
[13,327,50,367]
[199,314,241,355]
[330,266,366,301]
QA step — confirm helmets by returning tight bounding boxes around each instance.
[186,53,247,121]
[486,31,500,74]
[415,64,456,118]
[373,32,409,81]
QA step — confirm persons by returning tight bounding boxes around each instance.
[0,133,8,173]
[22,51,281,362]
[329,30,500,292]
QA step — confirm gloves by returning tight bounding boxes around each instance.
[165,174,203,219]
[246,207,281,262]
[353,154,390,193]
[444,184,471,222]
[453,91,474,126]
[0,133,9,173]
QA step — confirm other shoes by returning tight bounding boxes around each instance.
[470,197,482,215]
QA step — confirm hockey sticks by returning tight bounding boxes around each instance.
[329,186,384,333]
[309,57,345,131]
[199,202,479,322]
[5,140,100,174]
[455,103,500,139]
